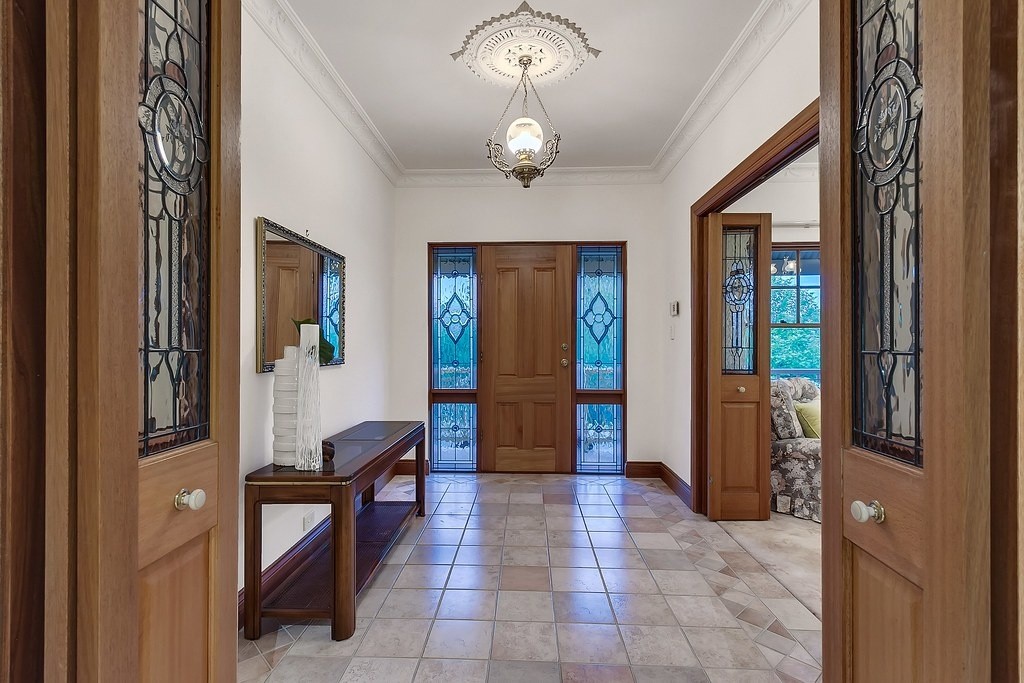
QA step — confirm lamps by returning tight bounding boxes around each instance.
[771,249,804,287]
[451,1,600,189]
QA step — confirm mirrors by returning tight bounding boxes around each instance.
[257,217,346,372]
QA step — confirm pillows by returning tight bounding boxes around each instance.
[795,398,821,439]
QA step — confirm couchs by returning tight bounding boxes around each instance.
[770,377,821,523]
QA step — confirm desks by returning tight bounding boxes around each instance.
[246,420,424,642]
